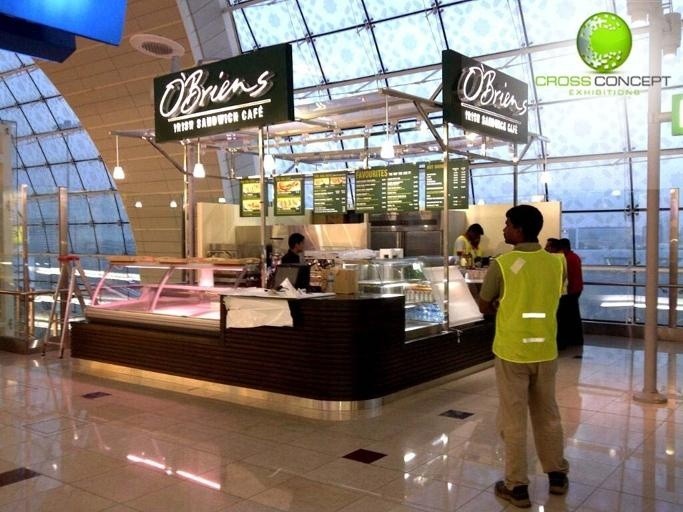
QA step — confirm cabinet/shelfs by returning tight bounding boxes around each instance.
[341,256,447,341]
[85,260,260,338]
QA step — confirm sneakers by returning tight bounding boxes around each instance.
[548,471,570,494]
[493,480,531,508]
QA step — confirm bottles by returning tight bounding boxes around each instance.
[327,272,334,292]
[404,285,434,303]
[405,303,444,323]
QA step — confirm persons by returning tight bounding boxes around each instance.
[558,239,584,345]
[279,233,305,264]
[478,205,569,507]
[544,238,569,352]
[452,224,484,258]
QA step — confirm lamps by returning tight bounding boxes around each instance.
[193,137,206,178]
[263,126,274,170]
[113,135,125,180]
[380,94,395,159]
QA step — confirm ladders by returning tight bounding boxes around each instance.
[41,254,100,359]
